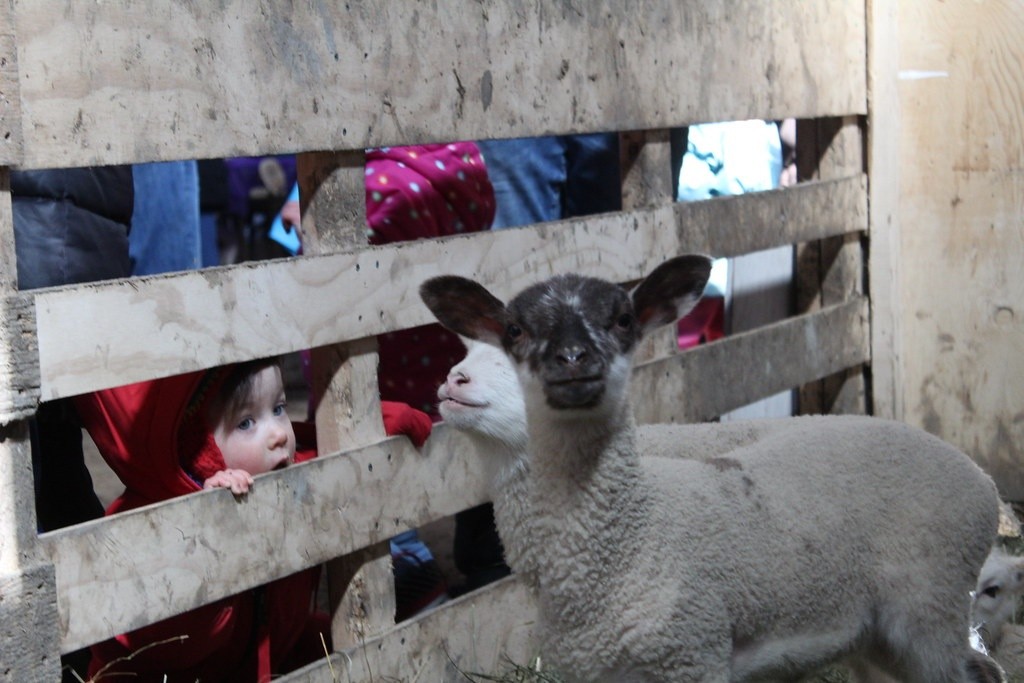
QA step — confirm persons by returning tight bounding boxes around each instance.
[80,355,431,683]
[17,108,799,530]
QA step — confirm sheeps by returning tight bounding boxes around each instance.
[417,254,1007,683]
[967,544,1024,683]
[437,334,847,599]
